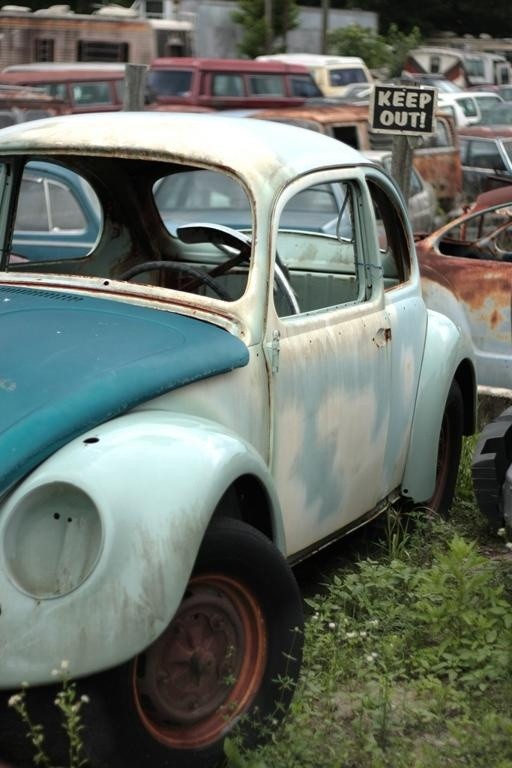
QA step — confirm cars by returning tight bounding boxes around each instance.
[0,112,479,766]
